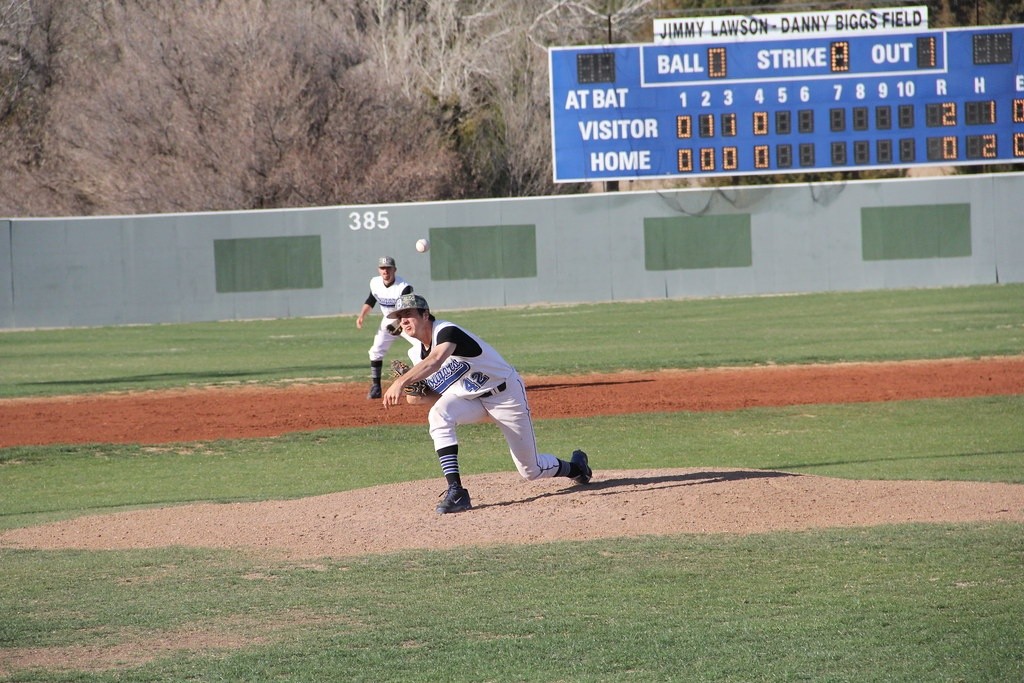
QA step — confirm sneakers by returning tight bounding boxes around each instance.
[572,449,592,484]
[368,387,382,398]
[436,488,473,515]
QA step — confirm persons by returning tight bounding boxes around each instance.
[382,294,592,514]
[356,257,420,399]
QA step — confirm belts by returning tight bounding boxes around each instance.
[479,382,506,398]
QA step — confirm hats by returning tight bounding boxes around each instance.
[379,256,395,268]
[386,295,430,319]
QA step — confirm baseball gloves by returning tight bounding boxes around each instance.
[389,360,429,398]
[386,319,402,335]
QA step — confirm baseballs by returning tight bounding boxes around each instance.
[415,239,430,253]
